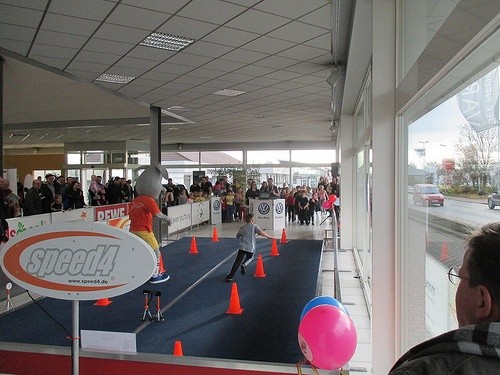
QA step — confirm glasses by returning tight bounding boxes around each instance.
[446,267,472,285]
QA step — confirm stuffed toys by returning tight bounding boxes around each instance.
[129,163,173,284]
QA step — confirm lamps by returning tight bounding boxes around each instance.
[327,64,340,88]
[329,119,338,132]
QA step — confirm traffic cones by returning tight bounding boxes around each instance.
[439,241,448,261]
[226,283,244,314]
[254,254,266,277]
[94,298,112,306]
[210,227,220,242]
[270,239,279,256]
[159,257,167,274]
[173,341,183,357]
[188,236,199,254]
[280,229,288,243]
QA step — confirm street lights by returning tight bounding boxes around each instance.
[419,141,429,149]
[440,144,446,194]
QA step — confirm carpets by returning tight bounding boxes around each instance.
[0,236,324,366]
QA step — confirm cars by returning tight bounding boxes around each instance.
[414,184,444,207]
[487,191,500,209]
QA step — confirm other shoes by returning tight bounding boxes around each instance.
[150,273,170,284]
[240,264,246,276]
[225,276,234,282]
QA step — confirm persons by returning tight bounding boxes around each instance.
[50,193,64,212]
[225,212,273,282]
[66,181,87,211]
[246,181,261,205]
[0,169,340,230]
[387,220,500,375]
[266,177,279,198]
[23,179,50,215]
